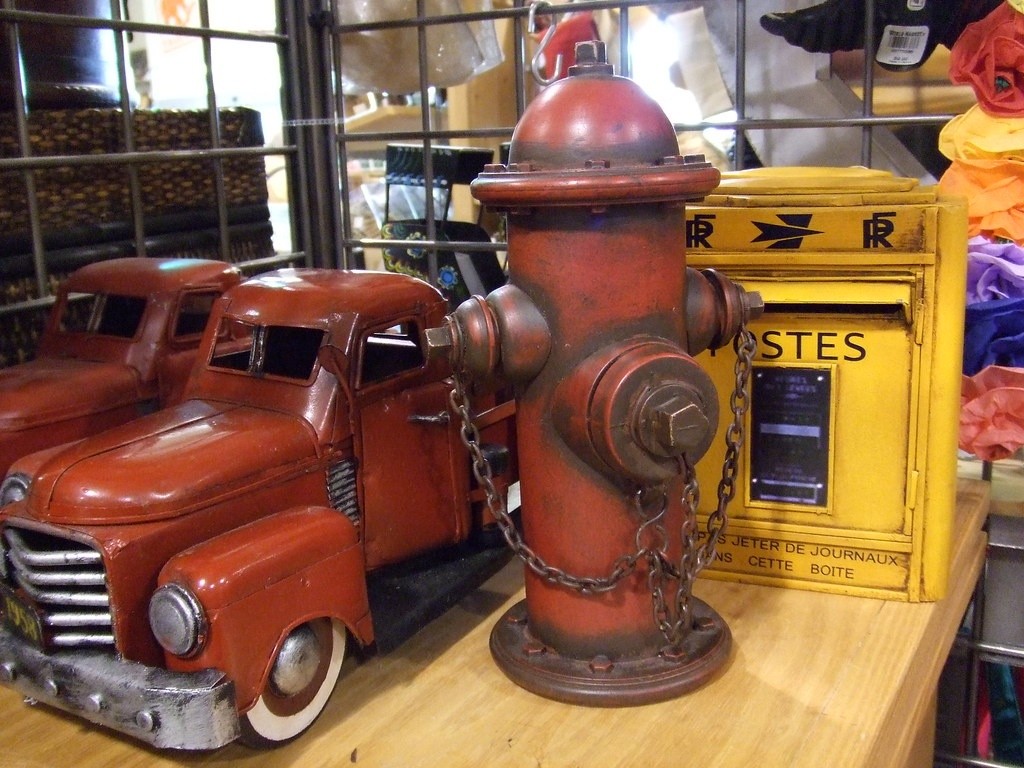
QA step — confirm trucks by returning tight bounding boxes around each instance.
[2,257,254,487]
[1,268,521,754]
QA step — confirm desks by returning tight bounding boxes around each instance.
[0,479,995,768]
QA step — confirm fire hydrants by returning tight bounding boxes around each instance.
[414,32,773,711]
